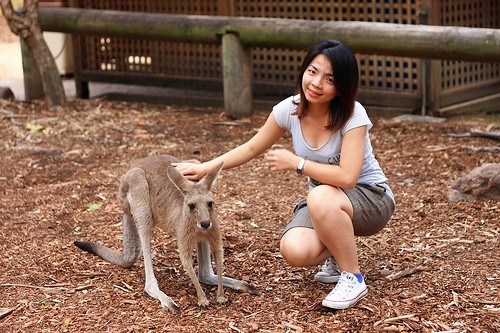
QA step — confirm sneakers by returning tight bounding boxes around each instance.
[314,257,342,283]
[322,270,367,311]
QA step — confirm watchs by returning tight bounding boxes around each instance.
[296,158,305,177]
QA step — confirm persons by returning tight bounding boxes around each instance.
[170,40,396,309]
